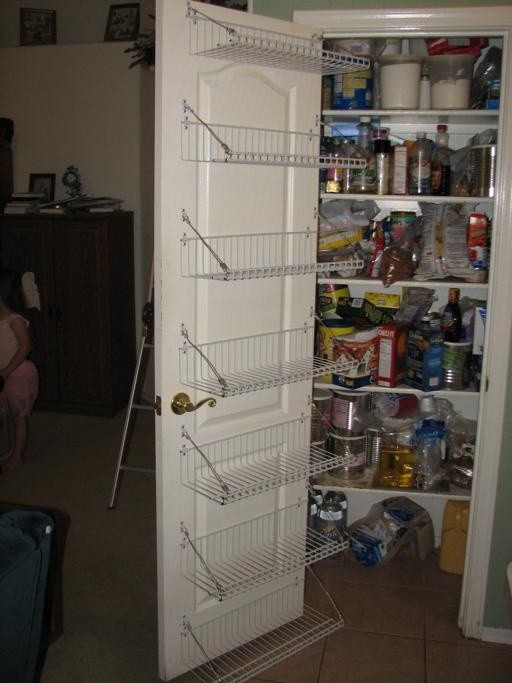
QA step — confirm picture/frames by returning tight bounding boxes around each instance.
[30,173,55,200]
[205,0,252,13]
[20,8,56,45]
[104,4,139,41]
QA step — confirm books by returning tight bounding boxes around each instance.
[3,192,73,215]
[89,206,114,213]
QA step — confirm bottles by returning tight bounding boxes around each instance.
[439,287,465,342]
[369,432,384,465]
[308,489,347,540]
[327,114,452,197]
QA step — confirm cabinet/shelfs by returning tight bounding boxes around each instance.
[154,2,511,682]
[0,211,135,417]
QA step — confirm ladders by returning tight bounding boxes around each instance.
[108,258,156,510]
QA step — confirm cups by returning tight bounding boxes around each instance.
[444,341,473,389]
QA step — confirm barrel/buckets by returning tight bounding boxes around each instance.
[439,500,470,576]
[308,488,348,558]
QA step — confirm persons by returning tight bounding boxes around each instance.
[0,274,40,471]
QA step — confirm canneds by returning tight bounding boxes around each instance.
[442,339,473,391]
[471,144,495,199]
[390,210,415,245]
[333,390,371,435]
[311,387,333,442]
[318,320,356,383]
[317,283,350,313]
[328,430,369,478]
[367,427,391,468]
[310,433,330,484]
